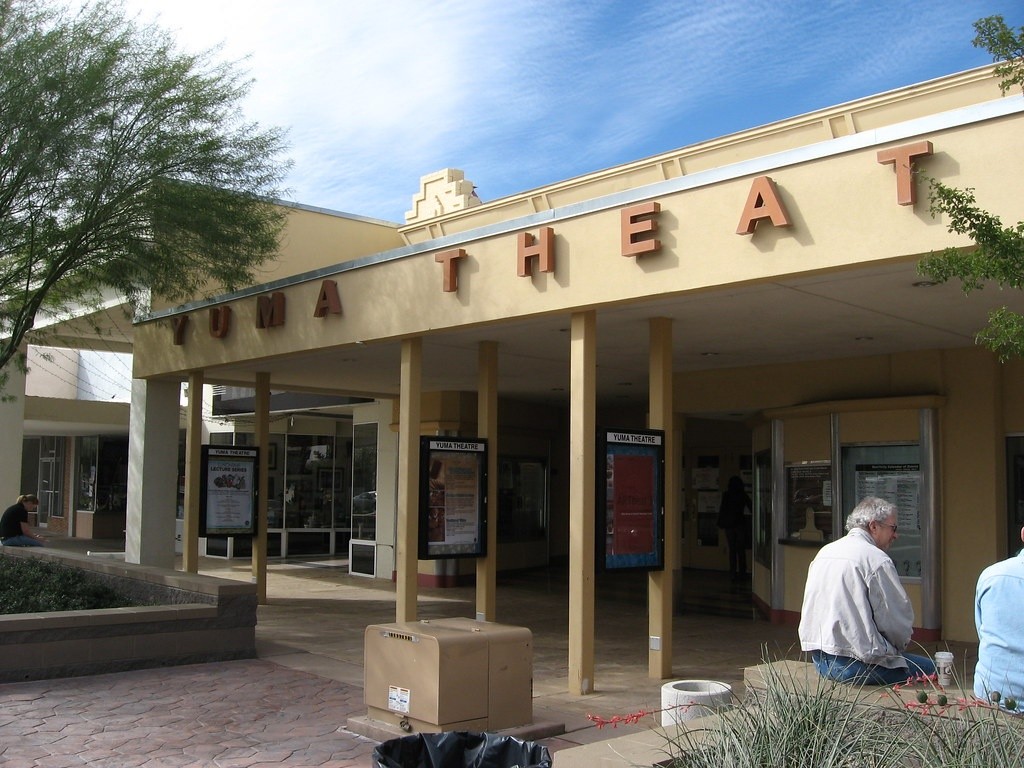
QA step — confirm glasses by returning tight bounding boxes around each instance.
[876,520,897,533]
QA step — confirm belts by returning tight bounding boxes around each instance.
[0,537,9,541]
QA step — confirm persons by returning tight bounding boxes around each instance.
[795,496,932,688]
[0,493,46,549]
[972,521,1024,717]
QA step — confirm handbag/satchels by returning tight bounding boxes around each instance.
[716,493,727,530]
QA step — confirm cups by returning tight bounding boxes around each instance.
[934,652,954,685]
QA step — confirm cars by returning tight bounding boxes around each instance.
[266,499,283,527]
[320,486,350,510]
[353,490,377,514]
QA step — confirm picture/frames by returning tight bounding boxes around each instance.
[317,437,336,459]
[317,467,345,492]
[284,451,312,474]
[268,443,276,469]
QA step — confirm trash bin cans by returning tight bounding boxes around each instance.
[369,729,554,768]
